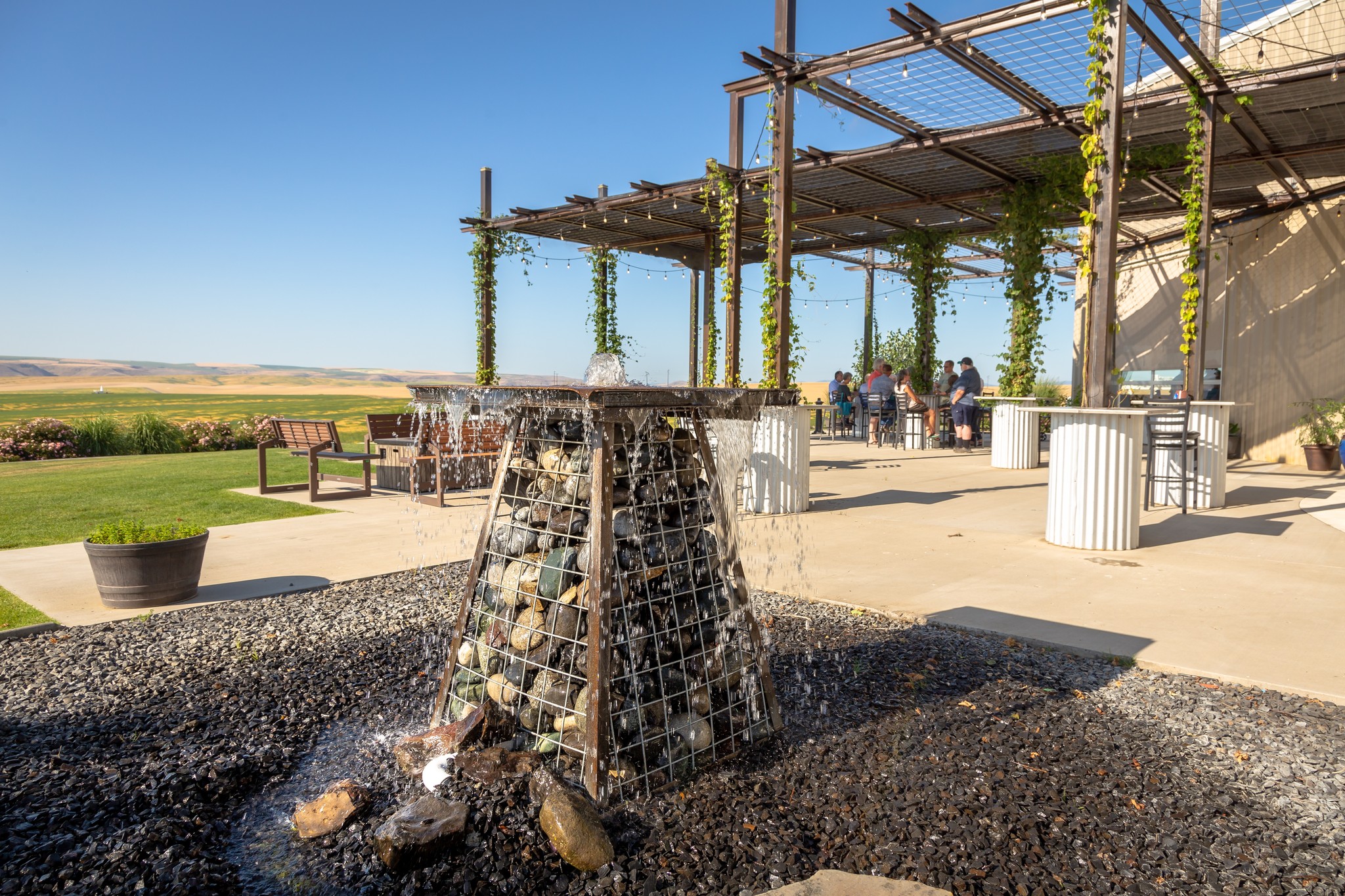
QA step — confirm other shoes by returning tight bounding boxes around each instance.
[880,423,891,433]
[940,440,957,445]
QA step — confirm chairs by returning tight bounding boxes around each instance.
[939,409,957,449]
[893,391,925,451]
[831,391,856,441]
[859,392,869,440]
[1139,395,1202,516]
[972,392,995,449]
[866,392,901,449]
[256,417,381,503]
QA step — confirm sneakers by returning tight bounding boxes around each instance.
[953,447,973,453]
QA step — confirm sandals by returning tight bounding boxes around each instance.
[926,433,941,441]
[978,442,982,446]
[970,444,976,447]
[849,420,857,427]
[840,420,847,424]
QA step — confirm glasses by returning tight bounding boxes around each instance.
[909,373,910,376]
[960,364,962,366]
[943,366,948,368]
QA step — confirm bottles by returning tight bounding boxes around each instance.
[853,378,857,392]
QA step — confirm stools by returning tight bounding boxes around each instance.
[818,408,848,442]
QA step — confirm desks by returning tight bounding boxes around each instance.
[851,395,870,438]
[973,395,1059,471]
[743,404,840,515]
[903,394,942,449]
[1129,399,1236,509]
[1017,405,1181,552]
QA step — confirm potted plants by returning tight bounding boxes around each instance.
[1227,423,1241,459]
[82,517,210,610]
[1287,397,1345,472]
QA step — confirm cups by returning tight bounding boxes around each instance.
[932,386,938,395]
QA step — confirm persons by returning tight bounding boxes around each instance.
[858,373,871,409]
[829,371,859,427]
[889,366,941,441]
[968,366,984,449]
[934,360,960,445]
[867,358,886,444]
[949,357,981,452]
[870,365,902,432]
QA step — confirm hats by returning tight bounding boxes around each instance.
[957,357,973,366]
[882,365,892,371]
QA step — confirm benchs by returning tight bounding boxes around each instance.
[364,411,521,493]
[397,419,528,508]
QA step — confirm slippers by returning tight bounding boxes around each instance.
[869,440,879,444]
